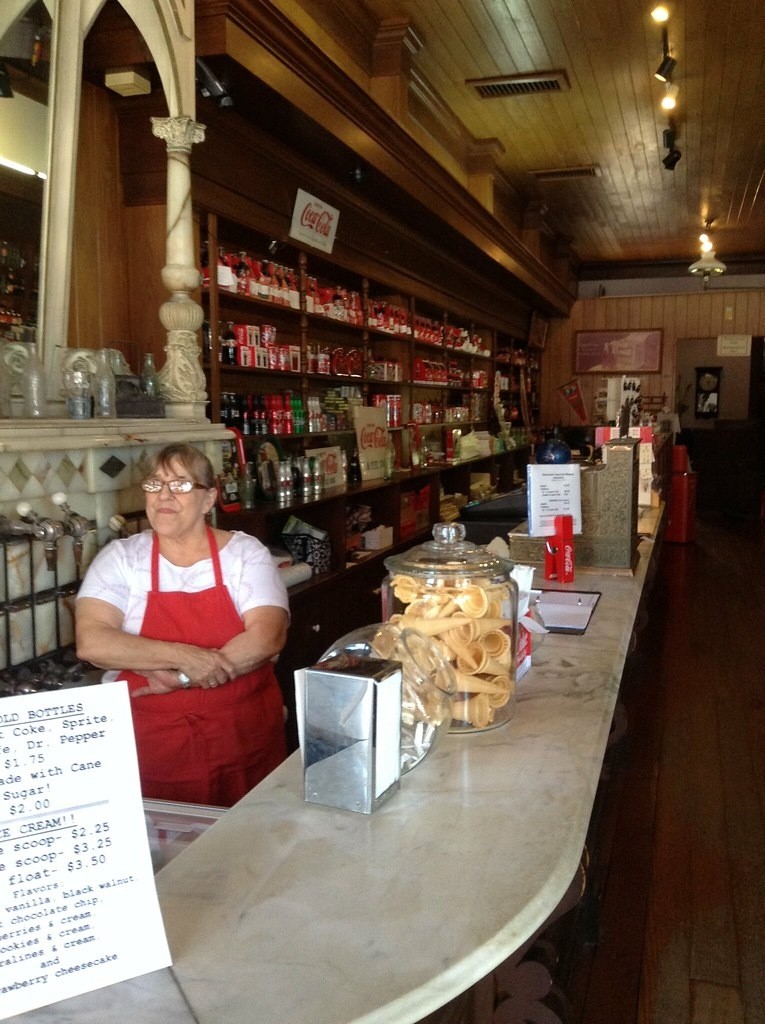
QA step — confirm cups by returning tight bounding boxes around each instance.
[62,371,93,419]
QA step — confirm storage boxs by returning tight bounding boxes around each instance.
[510,565,550,685]
[346,530,361,552]
[401,483,430,541]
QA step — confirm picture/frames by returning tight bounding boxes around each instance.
[572,327,664,375]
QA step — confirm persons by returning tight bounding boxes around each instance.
[76,444,291,809]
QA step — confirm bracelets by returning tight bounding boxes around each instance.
[179,670,192,690]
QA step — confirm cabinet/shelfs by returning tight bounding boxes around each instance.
[306,251,367,437]
[214,425,400,602]
[402,415,543,551]
[366,279,413,430]
[414,294,446,424]
[274,533,435,755]
[447,307,475,424]
[476,320,540,421]
[198,208,307,438]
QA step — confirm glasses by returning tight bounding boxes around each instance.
[136,477,208,496]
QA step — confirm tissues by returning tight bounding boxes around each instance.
[366,525,393,550]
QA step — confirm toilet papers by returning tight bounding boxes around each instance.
[277,562,313,588]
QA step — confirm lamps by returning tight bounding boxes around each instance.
[688,250,726,282]
[655,27,682,171]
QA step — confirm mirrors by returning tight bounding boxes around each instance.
[0,164,44,342]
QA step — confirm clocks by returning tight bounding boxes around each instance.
[694,366,723,421]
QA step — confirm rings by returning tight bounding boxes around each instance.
[209,680,217,686]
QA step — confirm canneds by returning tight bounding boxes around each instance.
[306,353,331,375]
[259,324,290,370]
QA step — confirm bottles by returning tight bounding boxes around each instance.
[367,299,412,337]
[112,352,126,375]
[507,428,527,445]
[305,272,362,325]
[341,450,347,481]
[453,429,461,460]
[28,343,48,418]
[238,451,321,509]
[307,343,364,378]
[142,353,160,396]
[383,434,396,480]
[220,388,303,435]
[350,448,360,482]
[414,315,442,345]
[218,321,223,363]
[200,240,296,308]
[419,433,427,467]
[94,348,116,418]
[307,395,335,432]
[554,514,574,582]
[223,322,237,364]
